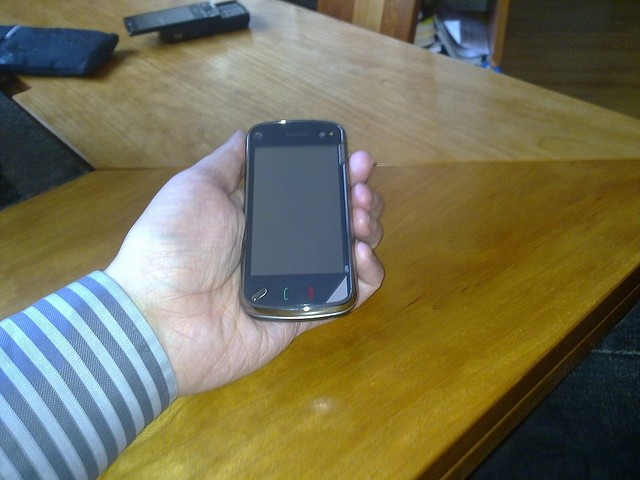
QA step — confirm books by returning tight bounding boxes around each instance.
[411,6,501,59]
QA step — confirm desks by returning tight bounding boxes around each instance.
[0,1,639,479]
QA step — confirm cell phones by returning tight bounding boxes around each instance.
[239,120,359,322]
[123,0,250,43]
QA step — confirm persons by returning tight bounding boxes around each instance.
[0,130,386,479]
